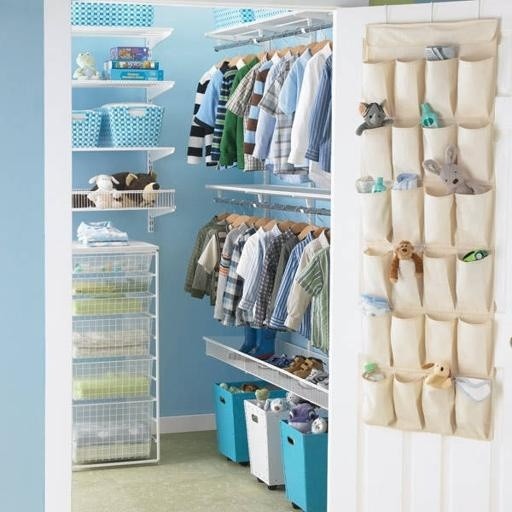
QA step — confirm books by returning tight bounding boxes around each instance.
[102,46,164,81]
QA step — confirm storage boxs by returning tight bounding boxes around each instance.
[213,380,288,467]
[279,419,328,512]
[243,399,289,490]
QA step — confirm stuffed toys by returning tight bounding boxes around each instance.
[355,100,394,137]
[92,170,160,206]
[389,240,423,284]
[421,144,491,194]
[422,361,453,389]
[87,174,122,210]
[72,52,100,80]
[219,383,327,434]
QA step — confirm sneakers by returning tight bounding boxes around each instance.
[318,378,328,388]
[283,355,304,372]
[273,354,292,368]
[266,351,280,362]
[305,366,328,383]
[293,357,324,378]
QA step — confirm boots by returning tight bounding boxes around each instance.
[239,325,256,352]
[250,328,276,360]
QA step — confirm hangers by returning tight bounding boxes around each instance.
[214,197,330,247]
[213,22,333,69]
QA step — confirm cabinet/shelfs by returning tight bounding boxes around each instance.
[70,240,161,472]
[70,23,178,234]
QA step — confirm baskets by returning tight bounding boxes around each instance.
[213,9,255,26]
[70,110,102,148]
[255,9,286,19]
[98,107,163,147]
[71,2,154,27]
